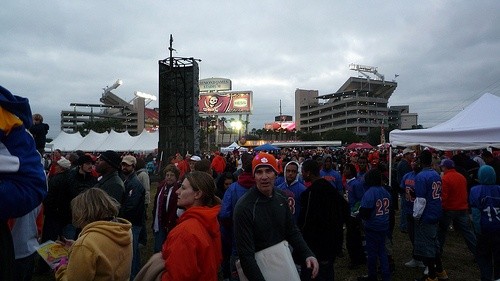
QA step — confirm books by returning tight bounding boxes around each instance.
[36,239,68,270]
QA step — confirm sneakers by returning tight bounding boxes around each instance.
[360,272,368,279]
[416,269,448,281]
[405,260,436,274]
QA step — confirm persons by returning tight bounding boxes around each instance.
[0,87,499,280]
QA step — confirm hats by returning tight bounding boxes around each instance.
[99,150,121,170]
[57,159,72,170]
[190,155,202,162]
[251,153,280,177]
[76,155,97,164]
[121,155,137,165]
[439,159,454,168]
[403,148,415,154]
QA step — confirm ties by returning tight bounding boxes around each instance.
[160,184,173,227]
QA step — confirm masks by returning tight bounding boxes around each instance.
[325,162,330,168]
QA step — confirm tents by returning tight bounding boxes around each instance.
[388,92,500,187]
[220,142,248,152]
